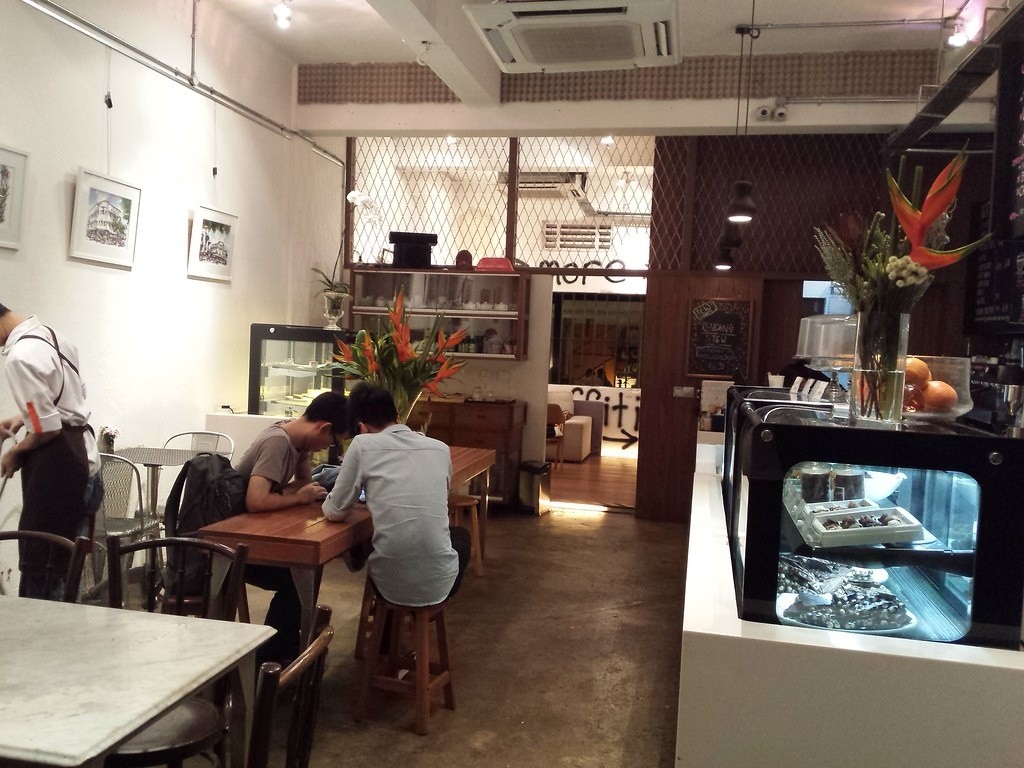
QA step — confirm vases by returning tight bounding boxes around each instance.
[849,312,910,430]
[323,292,348,331]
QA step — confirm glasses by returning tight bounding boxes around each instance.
[330,430,337,448]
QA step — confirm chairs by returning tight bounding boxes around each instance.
[0,431,332,768]
[546,403,566,473]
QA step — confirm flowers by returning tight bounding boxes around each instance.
[814,140,996,419]
[310,191,372,318]
[323,283,473,424]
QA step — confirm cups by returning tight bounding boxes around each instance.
[472,386,495,402]
[769,376,785,387]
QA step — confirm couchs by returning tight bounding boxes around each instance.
[547,391,592,461]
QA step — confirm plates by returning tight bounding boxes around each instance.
[286,396,309,401]
[849,564,888,585]
[775,592,917,636]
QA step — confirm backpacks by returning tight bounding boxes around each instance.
[165,451,245,594]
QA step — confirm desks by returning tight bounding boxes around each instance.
[89,447,231,604]
[1,594,279,768]
[205,444,495,709]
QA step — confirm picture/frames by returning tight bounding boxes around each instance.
[70,168,145,269]
[0,145,30,251]
[187,205,240,283]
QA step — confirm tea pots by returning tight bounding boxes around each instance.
[358,295,510,311]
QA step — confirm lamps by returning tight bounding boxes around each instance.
[274,0,293,28]
[943,17,969,47]
[714,0,758,271]
[616,171,636,187]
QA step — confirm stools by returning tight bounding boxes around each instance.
[448,493,484,578]
[357,570,455,734]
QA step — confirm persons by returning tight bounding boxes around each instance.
[597,368,613,387]
[0,303,105,602]
[322,382,471,654]
[575,368,598,386]
[234,392,350,675]
[483,328,512,355]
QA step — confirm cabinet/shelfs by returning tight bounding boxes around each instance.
[249,323,377,464]
[718,384,1024,651]
[408,400,527,510]
[347,264,531,362]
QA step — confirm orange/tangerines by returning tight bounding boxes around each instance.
[855,357,958,412]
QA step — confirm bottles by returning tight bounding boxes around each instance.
[442,333,476,352]
[802,460,865,503]
